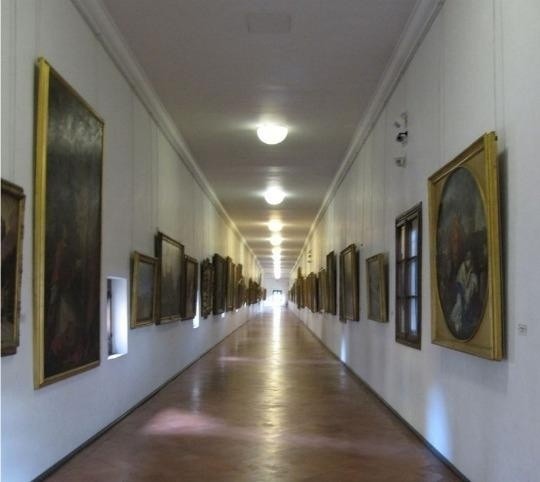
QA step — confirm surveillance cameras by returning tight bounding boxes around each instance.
[397,135,404,142]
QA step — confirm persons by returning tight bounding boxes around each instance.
[456,252,482,318]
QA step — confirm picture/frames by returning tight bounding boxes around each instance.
[130,230,265,330]
[366,253,388,322]
[0,177,25,357]
[288,244,359,323]
[33,58,105,393]
[428,129,503,361]
